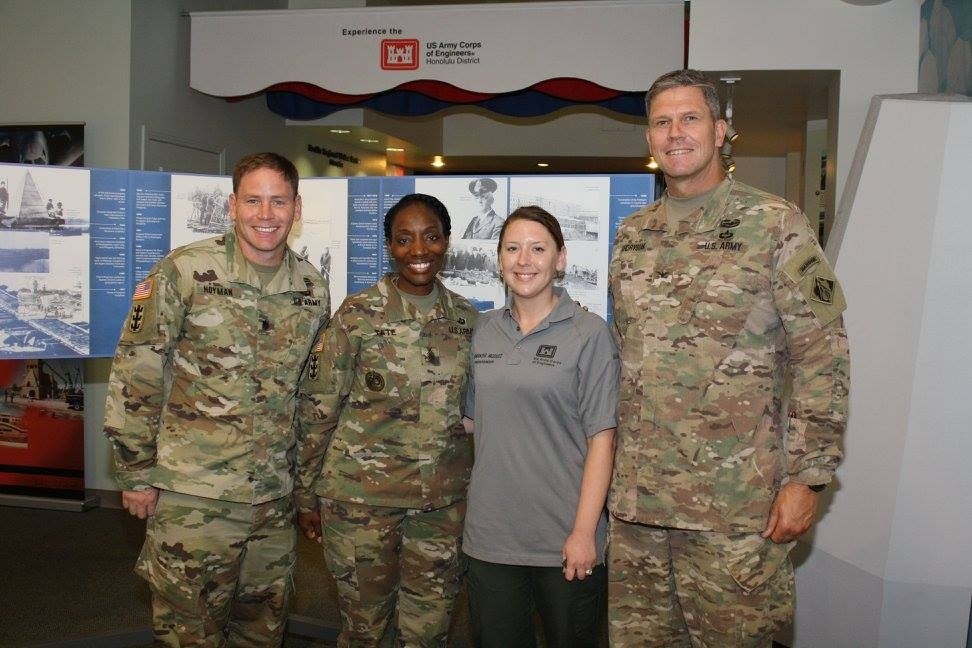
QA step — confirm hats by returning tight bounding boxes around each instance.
[468,178,498,196]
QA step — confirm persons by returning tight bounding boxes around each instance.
[288,194,477,648]
[461,205,616,648]
[105,150,329,648]
[604,69,851,648]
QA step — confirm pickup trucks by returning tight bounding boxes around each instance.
[65,394,84,410]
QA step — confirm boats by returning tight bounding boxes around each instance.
[185,218,229,234]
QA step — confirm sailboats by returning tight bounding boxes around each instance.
[0,169,66,228]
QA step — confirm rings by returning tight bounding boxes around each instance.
[585,569,592,575]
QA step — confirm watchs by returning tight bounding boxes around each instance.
[808,483,827,493]
